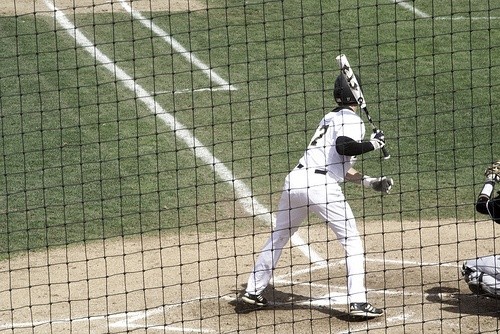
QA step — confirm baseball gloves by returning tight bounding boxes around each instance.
[486,161,500,184]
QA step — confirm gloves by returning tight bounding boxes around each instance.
[363,176,394,193]
[368,128,387,152]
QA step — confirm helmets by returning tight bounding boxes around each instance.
[334,71,363,108]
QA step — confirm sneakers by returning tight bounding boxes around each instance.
[347,302,384,318]
[239,292,270,306]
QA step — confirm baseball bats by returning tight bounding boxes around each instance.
[334,53,393,161]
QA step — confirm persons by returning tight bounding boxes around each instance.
[238,73,394,317]
[462,161,500,299]
